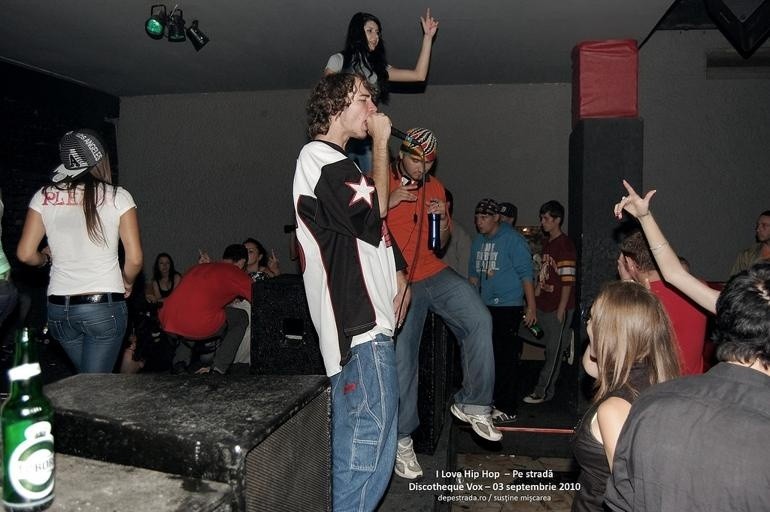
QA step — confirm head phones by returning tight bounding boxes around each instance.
[394,160,432,189]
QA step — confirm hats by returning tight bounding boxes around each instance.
[496,202,518,219]
[47,130,110,184]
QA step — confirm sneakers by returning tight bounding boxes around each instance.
[561,328,577,366]
[490,406,518,424]
[174,361,190,373]
[205,369,244,387]
[450,401,504,443]
[395,440,426,481]
[523,391,551,405]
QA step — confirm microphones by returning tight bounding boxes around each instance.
[365,111,423,149]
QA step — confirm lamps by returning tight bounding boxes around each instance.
[145,4,210,53]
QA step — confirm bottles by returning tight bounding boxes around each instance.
[427,200,441,251]
[519,311,544,340]
[0,326,57,512]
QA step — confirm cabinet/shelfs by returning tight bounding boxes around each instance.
[570,117,644,405]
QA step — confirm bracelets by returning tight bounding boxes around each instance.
[40,253,50,266]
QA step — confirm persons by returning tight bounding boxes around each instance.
[434,178,770,512]
[0,198,279,392]
[16,131,143,373]
[293,71,411,512]
[368,127,503,479]
[322,8,439,178]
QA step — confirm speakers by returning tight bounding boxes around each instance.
[394,310,456,457]
[250,270,325,376]
[1,443,233,512]
[45,369,335,511]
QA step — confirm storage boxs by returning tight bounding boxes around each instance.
[571,38,638,118]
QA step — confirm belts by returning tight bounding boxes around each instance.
[48,293,126,307]
